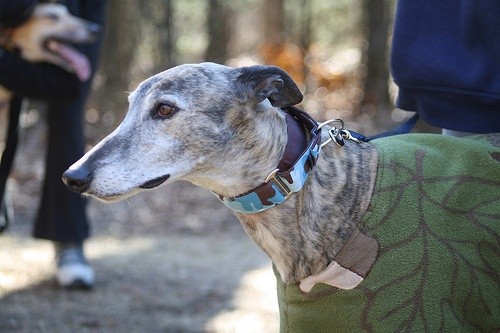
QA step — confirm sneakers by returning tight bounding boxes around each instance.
[54,238,94,286]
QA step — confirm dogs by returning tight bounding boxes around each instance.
[0,0,102,162]
[62,61,500,333]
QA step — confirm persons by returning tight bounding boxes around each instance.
[390,0,500,136]
[0,0,109,291]
